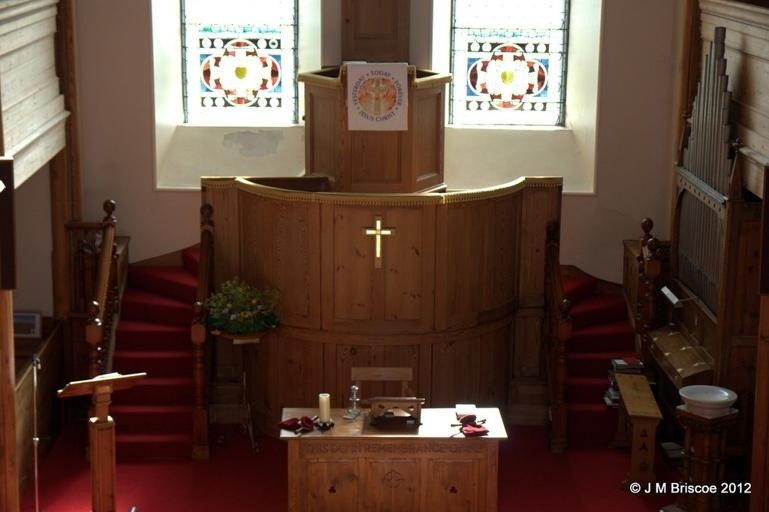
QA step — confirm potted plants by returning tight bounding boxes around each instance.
[200,275,280,329]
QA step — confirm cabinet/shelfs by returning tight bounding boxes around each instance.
[667,398,738,511]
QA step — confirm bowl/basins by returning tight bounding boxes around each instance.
[679,384,737,417]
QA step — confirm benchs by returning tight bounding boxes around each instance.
[607,367,665,494]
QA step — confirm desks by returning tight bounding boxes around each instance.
[280,397,510,510]
